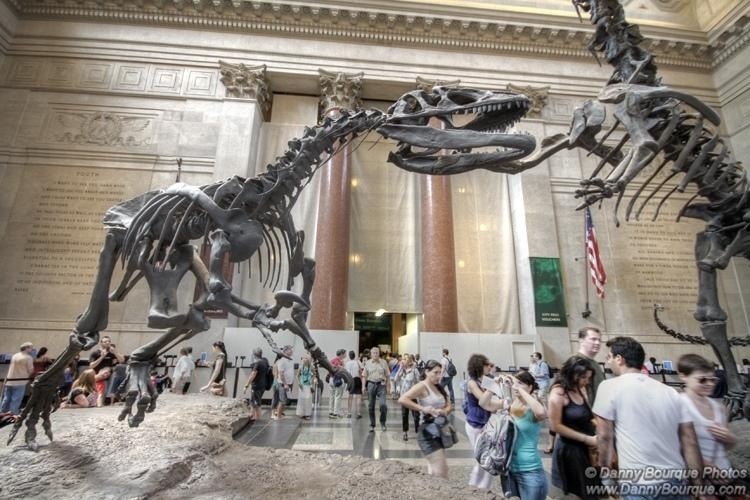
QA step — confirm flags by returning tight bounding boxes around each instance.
[584,202,607,305]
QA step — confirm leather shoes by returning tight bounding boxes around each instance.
[404,433,407,441]
[369,425,386,432]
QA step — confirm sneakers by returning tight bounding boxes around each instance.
[270,415,287,421]
[329,413,362,419]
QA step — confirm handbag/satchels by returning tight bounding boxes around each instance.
[440,422,458,448]
[211,382,224,396]
[334,377,342,387]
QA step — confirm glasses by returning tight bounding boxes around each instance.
[700,377,719,383]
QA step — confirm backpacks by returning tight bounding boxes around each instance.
[266,366,274,390]
[474,410,518,475]
[445,358,456,376]
[540,361,553,378]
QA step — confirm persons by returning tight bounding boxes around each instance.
[243,326,749,500]
[0,336,229,425]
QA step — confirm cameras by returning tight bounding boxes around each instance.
[500,373,513,390]
[105,344,115,352]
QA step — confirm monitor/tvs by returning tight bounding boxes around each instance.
[664,361,672,370]
